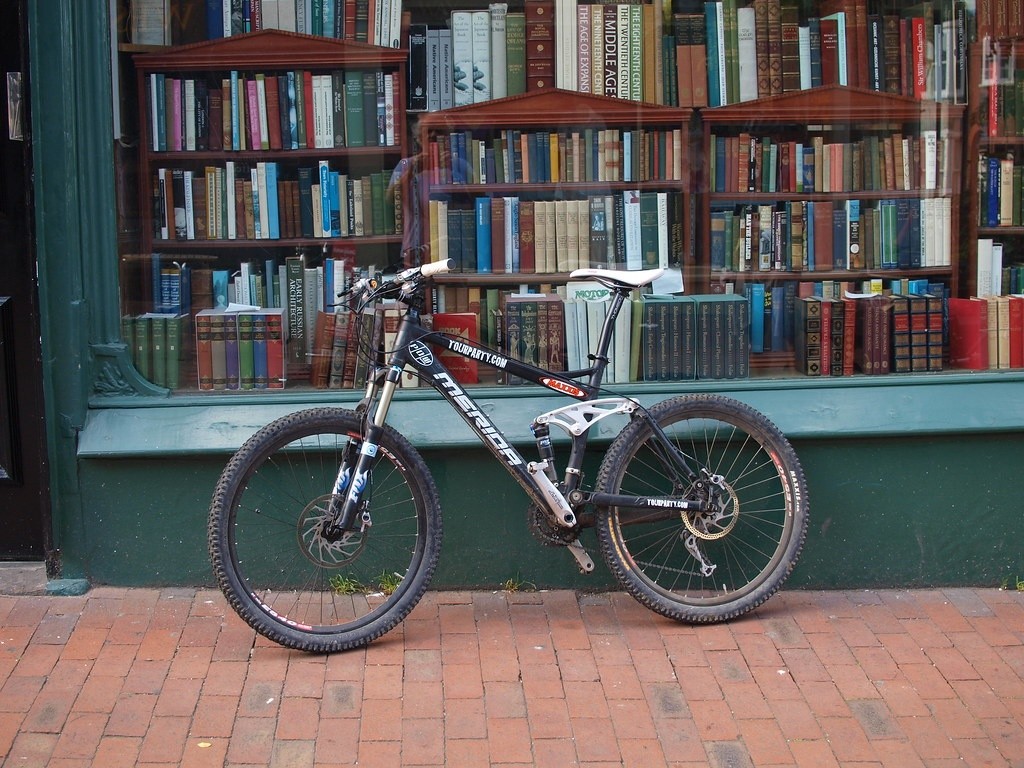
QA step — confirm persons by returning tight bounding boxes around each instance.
[386,115,479,313]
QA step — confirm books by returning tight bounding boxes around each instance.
[120,0,1024,390]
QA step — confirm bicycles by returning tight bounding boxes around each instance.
[203,258,807,655]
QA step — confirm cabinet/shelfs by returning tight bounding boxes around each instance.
[114,24,1024,370]
